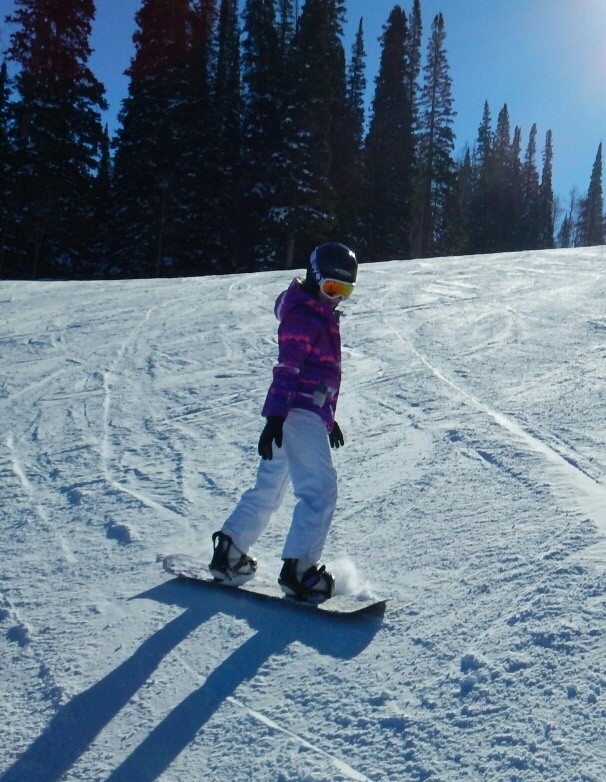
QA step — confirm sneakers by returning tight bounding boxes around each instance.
[279,559,337,602]
[208,532,258,585]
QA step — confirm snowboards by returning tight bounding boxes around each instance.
[161,553,397,616]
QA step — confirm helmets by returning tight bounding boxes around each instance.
[306,242,359,283]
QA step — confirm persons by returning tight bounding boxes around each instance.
[208,244,358,596]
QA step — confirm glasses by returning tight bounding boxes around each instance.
[320,278,355,302]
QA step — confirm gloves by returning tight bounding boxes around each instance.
[329,421,344,448]
[258,416,285,460]
